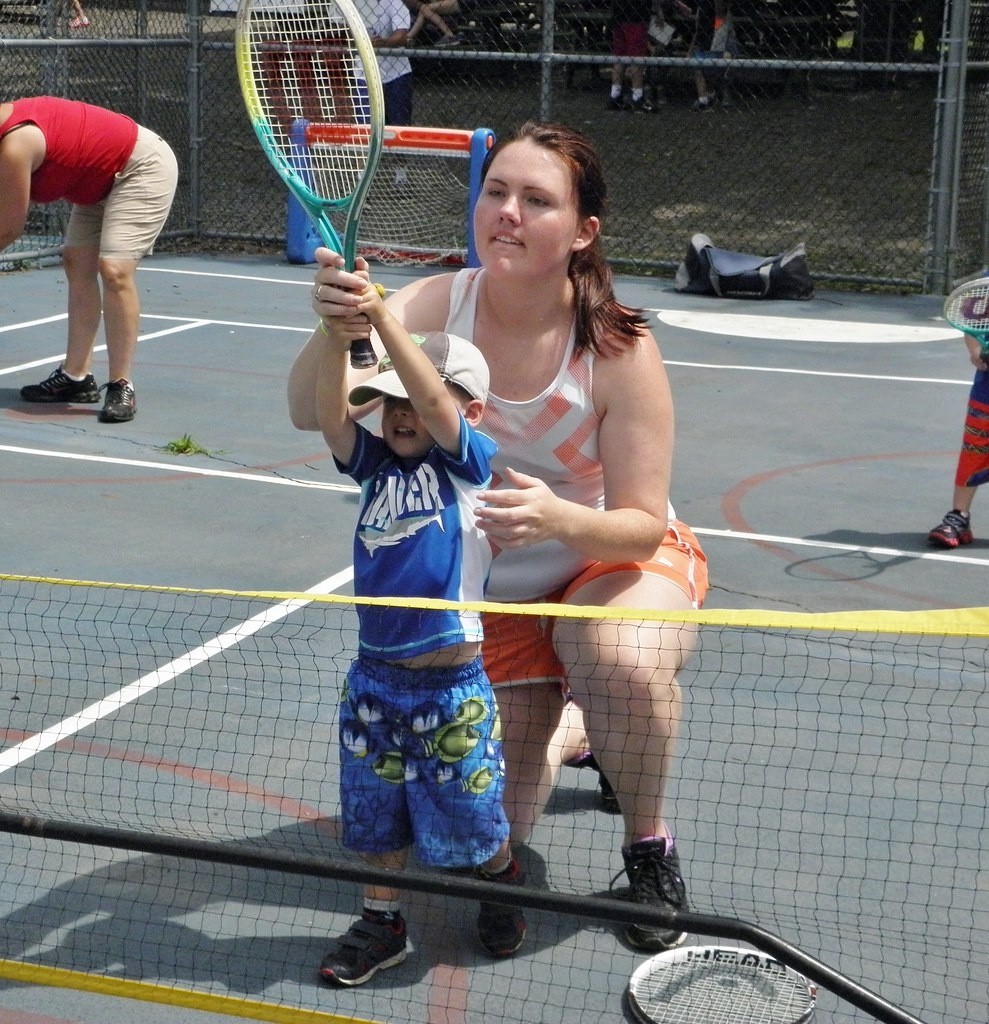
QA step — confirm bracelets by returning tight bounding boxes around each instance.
[320,319,328,336]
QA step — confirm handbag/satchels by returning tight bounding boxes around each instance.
[675,234,813,300]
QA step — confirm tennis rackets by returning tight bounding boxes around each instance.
[945,276,988,353]
[626,944,817,1024]
[234,0,390,370]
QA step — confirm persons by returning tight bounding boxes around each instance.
[288,119,710,951]
[0,95,178,422]
[405,0,475,47]
[320,269,525,991]
[927,268,989,549]
[52,0,90,28]
[327,0,413,218]
[603,0,737,114]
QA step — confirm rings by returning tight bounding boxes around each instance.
[315,286,323,301]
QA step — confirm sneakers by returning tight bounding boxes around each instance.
[320,912,407,985]
[20,361,101,402]
[609,837,689,951]
[99,378,137,419]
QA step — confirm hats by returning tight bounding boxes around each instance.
[349,332,489,407]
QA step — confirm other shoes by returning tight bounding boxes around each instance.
[563,692,620,814]
[929,509,972,547]
[689,98,717,114]
[477,860,526,954]
[605,94,662,116]
